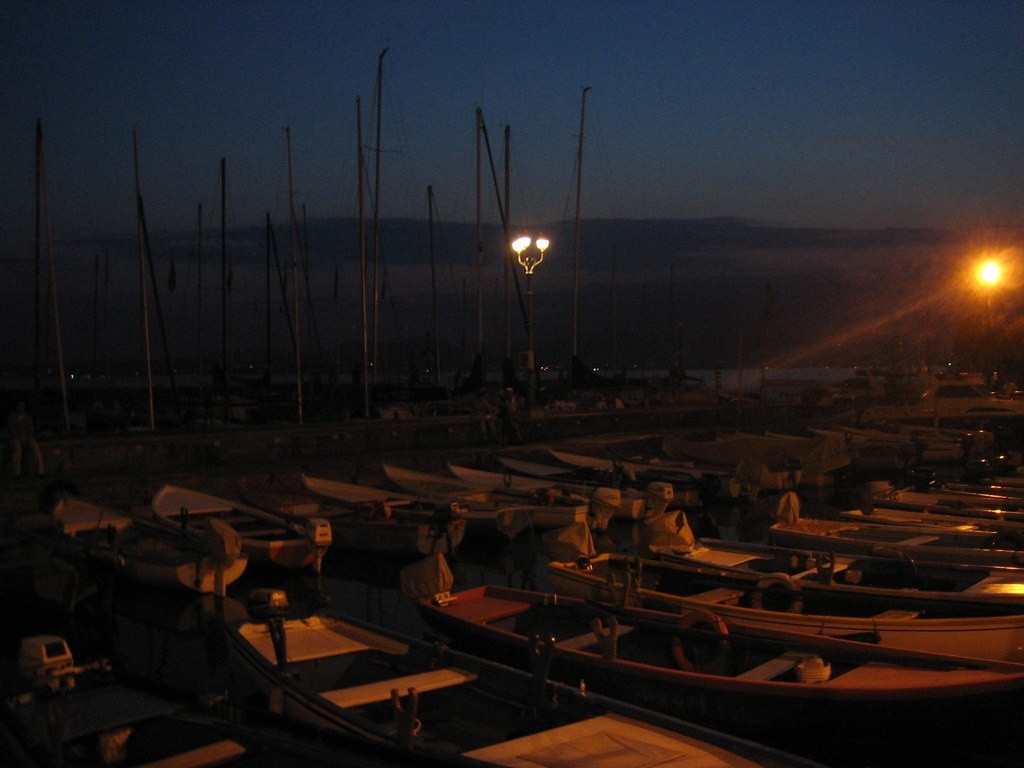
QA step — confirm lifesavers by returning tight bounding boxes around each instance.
[752,572,805,615]
[671,608,731,674]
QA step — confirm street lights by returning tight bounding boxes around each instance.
[511,236,550,416]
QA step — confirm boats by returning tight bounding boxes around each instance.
[0,44,1024,768]
